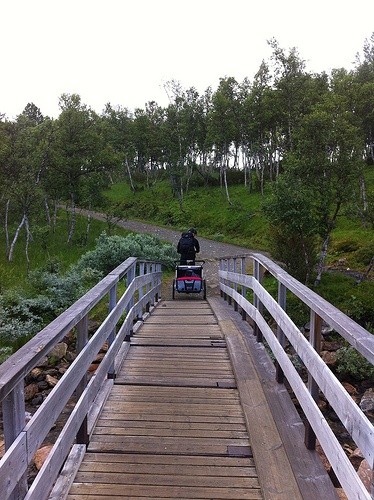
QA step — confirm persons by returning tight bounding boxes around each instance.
[177,228,200,267]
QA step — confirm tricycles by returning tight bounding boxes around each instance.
[172,250,206,301]
